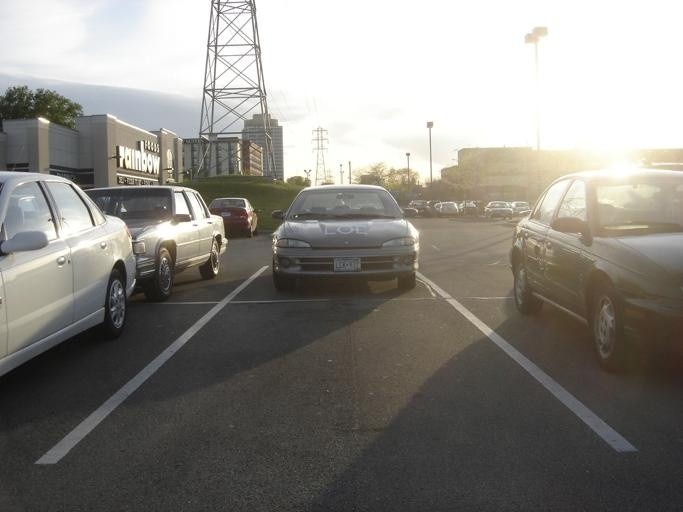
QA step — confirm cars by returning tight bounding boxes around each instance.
[402,199,531,220]
[0,171,137,381]
[208,197,257,237]
[270,185,419,290]
[82,185,228,300]
[510,166,683,371]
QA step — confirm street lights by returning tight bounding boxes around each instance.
[426,121,433,186]
[338,163,345,185]
[348,160,353,184]
[524,25,549,194]
[303,169,311,179]
[406,151,410,195]
